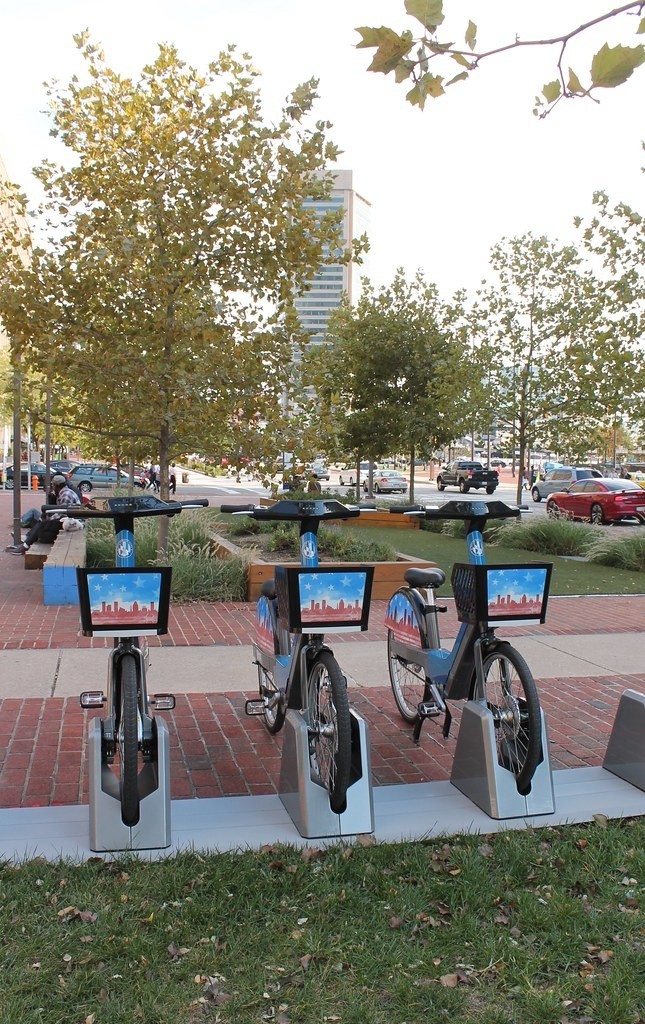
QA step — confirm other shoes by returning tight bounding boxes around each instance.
[11,544,28,555]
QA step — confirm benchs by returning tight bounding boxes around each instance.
[41,524,86,605]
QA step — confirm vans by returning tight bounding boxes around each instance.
[339,463,378,485]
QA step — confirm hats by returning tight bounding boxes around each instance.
[51,476,66,485]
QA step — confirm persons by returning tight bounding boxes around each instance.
[308,474,321,492]
[140,459,176,494]
[522,465,545,490]
[289,476,304,492]
[498,464,502,472]
[603,467,631,479]
[12,472,82,554]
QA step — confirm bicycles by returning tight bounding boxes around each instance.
[385,500,554,793]
[40,496,211,828]
[220,499,376,814]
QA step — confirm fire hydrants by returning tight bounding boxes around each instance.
[31,475,39,490]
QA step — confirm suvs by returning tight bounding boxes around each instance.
[65,462,145,493]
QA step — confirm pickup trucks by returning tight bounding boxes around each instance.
[436,461,499,495]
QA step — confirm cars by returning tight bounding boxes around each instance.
[532,466,603,502]
[310,464,330,480]
[545,478,645,524]
[49,460,81,474]
[618,472,645,489]
[0,462,58,490]
[363,470,408,494]
[100,464,150,477]
